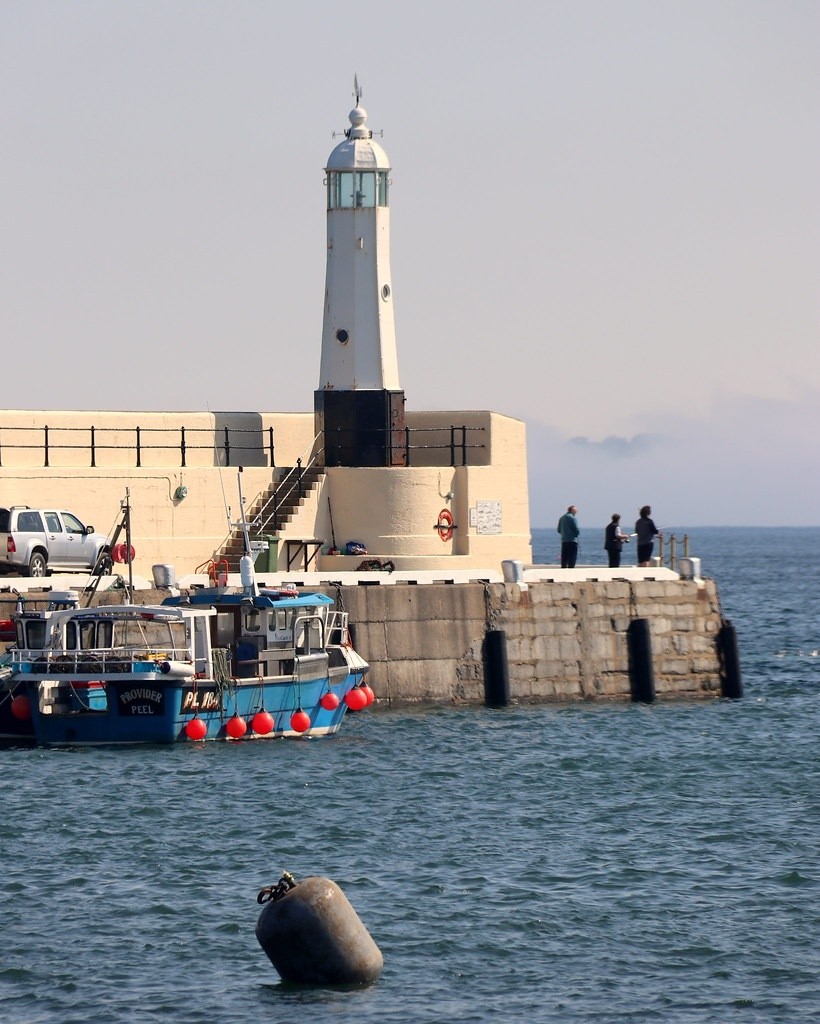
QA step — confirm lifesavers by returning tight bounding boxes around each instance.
[437,510,455,541]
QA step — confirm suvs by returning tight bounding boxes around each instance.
[0,505,115,577]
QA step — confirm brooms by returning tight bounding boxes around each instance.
[327,496,340,555]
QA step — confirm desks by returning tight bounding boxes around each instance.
[284,539,327,572]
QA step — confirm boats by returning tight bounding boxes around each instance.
[0,485,372,750]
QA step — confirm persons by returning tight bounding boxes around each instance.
[604,514,628,567]
[557,506,579,568]
[635,506,659,566]
